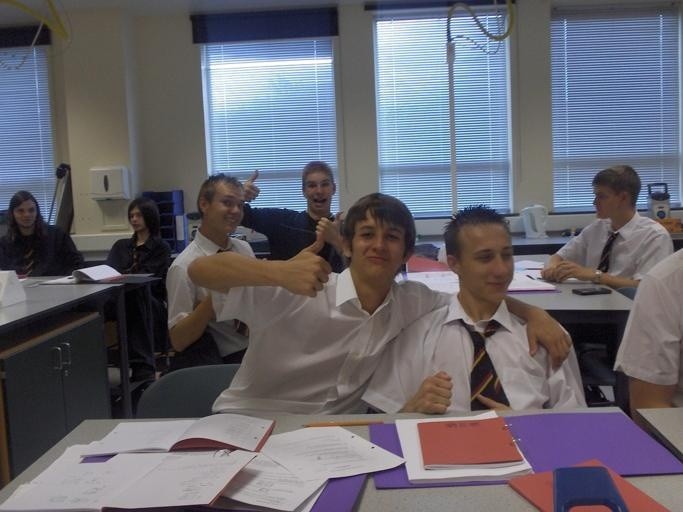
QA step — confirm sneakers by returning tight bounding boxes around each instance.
[105,346,157,389]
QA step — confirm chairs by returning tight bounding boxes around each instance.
[134,362,241,417]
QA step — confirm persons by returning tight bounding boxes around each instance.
[362,204,590,415]
[165,173,257,364]
[0,190,84,279]
[186,194,572,416]
[100,198,172,380]
[612,247,682,436]
[238,162,349,274]
[540,165,673,402]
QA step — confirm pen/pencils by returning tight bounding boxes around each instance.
[526,275,534,280]
[301,420,384,427]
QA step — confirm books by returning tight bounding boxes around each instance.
[415,415,527,470]
[80,413,275,454]
[39,262,123,286]
[509,457,669,511]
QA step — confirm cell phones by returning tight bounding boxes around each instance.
[572,287,612,295]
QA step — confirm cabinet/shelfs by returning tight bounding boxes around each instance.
[0,311,112,481]
[140,190,184,254]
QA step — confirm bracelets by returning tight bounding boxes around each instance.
[592,268,604,284]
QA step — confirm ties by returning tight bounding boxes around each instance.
[458,318,510,411]
[596,230,619,273]
[217,249,248,339]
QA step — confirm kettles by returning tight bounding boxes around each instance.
[520,205,550,239]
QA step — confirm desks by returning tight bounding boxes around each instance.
[407,232,683,320]
[1,268,164,418]
[0,408,683,512]
